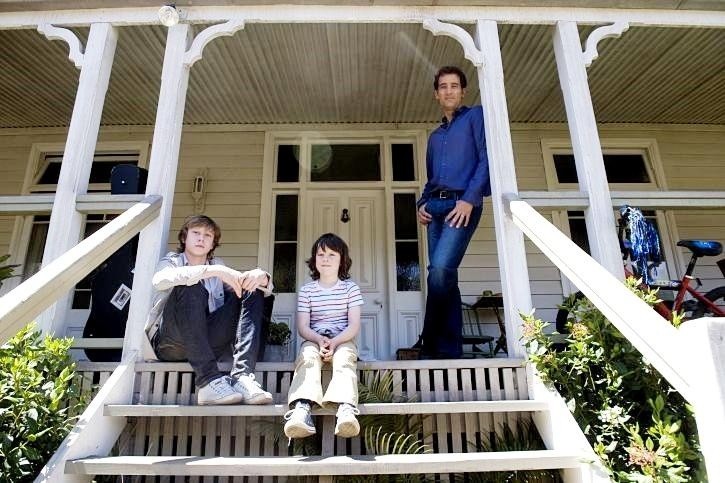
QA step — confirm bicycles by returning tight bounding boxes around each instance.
[556,240,725,334]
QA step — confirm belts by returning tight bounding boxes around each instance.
[432,190,460,200]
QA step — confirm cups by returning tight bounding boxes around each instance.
[483,291,493,296]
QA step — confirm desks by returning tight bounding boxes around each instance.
[470,297,507,355]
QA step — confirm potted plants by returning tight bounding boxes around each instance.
[263,322,292,362]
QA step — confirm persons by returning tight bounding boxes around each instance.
[143,213,278,406]
[282,230,366,441]
[413,65,493,360]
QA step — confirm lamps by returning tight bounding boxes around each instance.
[158,3,187,27]
[191,167,207,213]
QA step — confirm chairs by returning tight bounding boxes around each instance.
[461,302,494,358]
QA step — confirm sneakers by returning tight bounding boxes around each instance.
[284,401,316,438]
[334,403,360,437]
[197,373,273,405]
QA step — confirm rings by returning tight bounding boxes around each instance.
[461,214,466,217]
